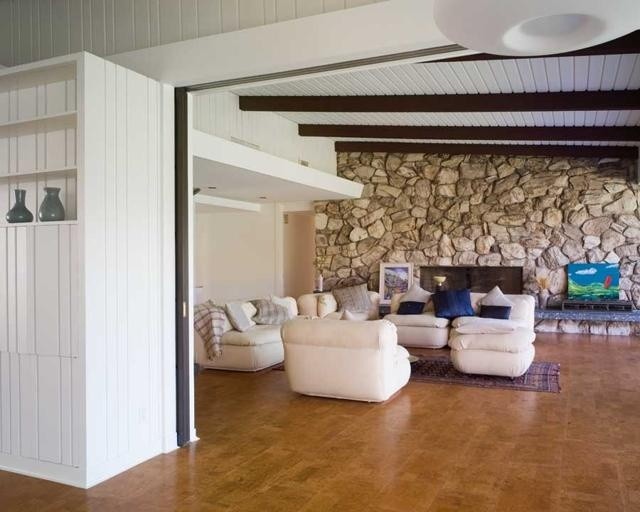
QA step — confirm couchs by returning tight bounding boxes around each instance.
[296,291,379,318]
[194,294,318,379]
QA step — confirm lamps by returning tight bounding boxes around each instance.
[430,0,640,57]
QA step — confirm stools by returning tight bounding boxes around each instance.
[450,332,535,381]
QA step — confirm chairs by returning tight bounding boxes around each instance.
[383,293,449,349]
[447,293,536,345]
[280,317,411,403]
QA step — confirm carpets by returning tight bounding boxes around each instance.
[271,354,564,394]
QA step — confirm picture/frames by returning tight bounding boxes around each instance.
[379,261,414,304]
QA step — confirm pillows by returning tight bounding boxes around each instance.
[225,282,515,334]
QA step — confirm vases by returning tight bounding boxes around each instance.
[6,189,33,223]
[38,187,65,221]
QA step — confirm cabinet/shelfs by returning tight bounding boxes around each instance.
[0,52,164,490]
[561,298,634,311]
